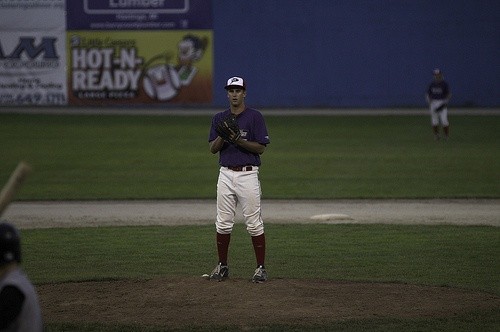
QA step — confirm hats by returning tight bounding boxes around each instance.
[224,76,246,89]
[431,68,442,75]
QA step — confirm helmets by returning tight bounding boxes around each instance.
[0,223,22,265]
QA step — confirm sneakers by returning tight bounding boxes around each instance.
[252,265,267,283]
[209,263,229,281]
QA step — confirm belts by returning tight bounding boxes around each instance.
[228,165,252,172]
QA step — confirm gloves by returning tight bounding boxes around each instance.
[219,119,239,140]
[215,122,242,144]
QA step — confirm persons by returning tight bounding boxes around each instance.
[206,77,270,284]
[0,220,42,332]
[425,69,452,143]
